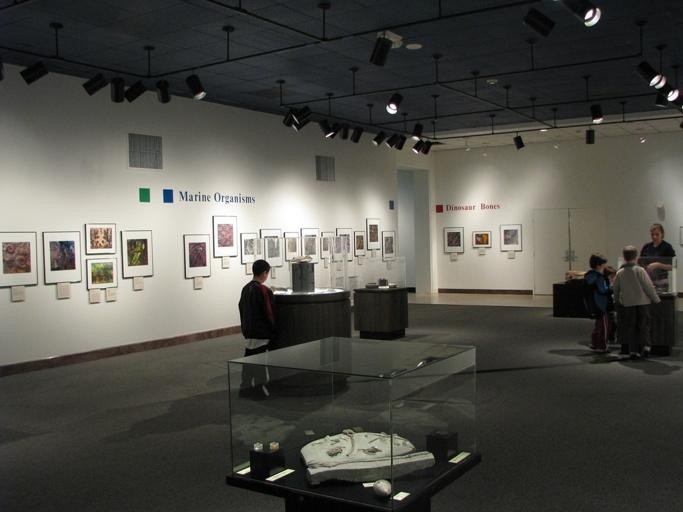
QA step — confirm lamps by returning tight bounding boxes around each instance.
[637,60,682,108]
[412,120,423,142]
[585,126,597,145]
[186,70,207,102]
[283,106,314,131]
[319,120,365,144]
[565,4,603,28]
[523,6,556,37]
[372,131,434,155]
[514,131,526,151]
[20,20,155,104]
[386,91,405,116]
[590,102,605,124]
[370,30,393,68]
[155,76,174,106]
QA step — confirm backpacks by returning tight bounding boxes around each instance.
[581,273,603,317]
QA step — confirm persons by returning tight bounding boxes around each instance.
[606,267,617,343]
[639,224,676,292]
[615,244,665,359]
[577,252,615,352]
[239,260,276,398]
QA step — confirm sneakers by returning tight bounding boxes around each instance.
[588,337,645,363]
[253,385,278,401]
[239,383,256,397]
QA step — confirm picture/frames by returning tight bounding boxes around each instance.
[43,231,82,284]
[383,231,397,261]
[1,232,38,287]
[500,224,521,251]
[121,229,153,279]
[213,216,239,258]
[443,227,465,253]
[366,218,381,250]
[86,223,117,255]
[86,258,119,290]
[240,228,352,267]
[184,234,212,278]
[472,231,492,248]
[354,231,367,257]
